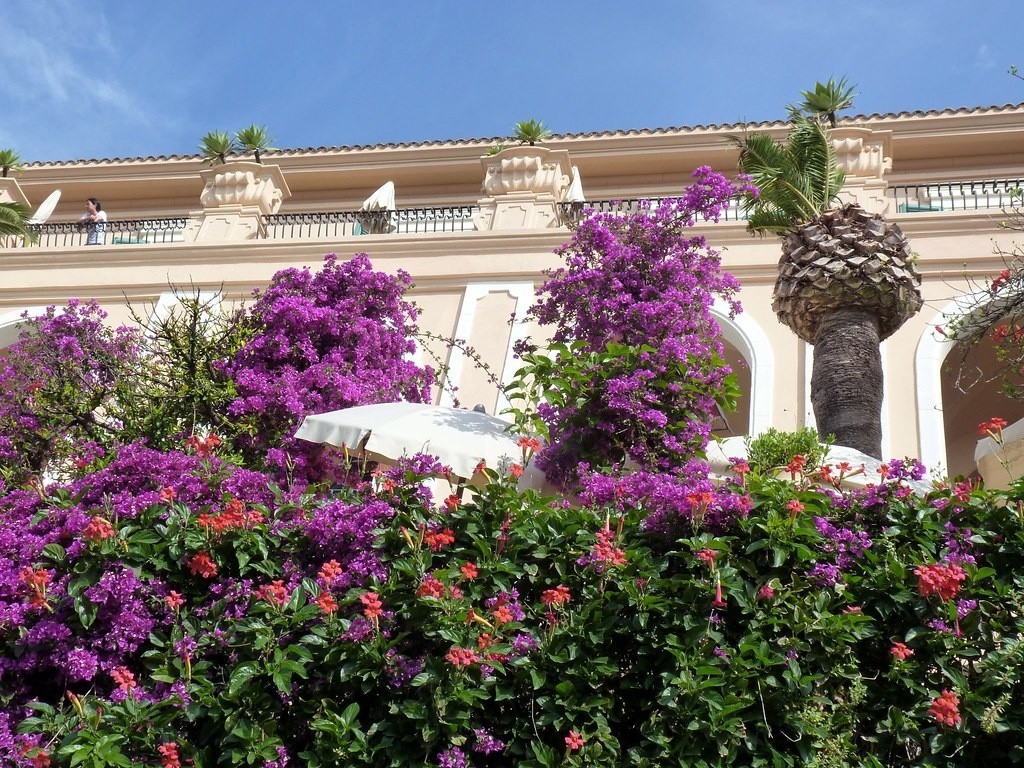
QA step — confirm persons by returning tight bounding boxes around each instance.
[75,197,107,244]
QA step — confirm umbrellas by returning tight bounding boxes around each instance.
[974,414,1024,507]
[614,434,936,500]
[18,188,62,247]
[356,179,398,235]
[562,164,587,219]
[292,402,560,504]
